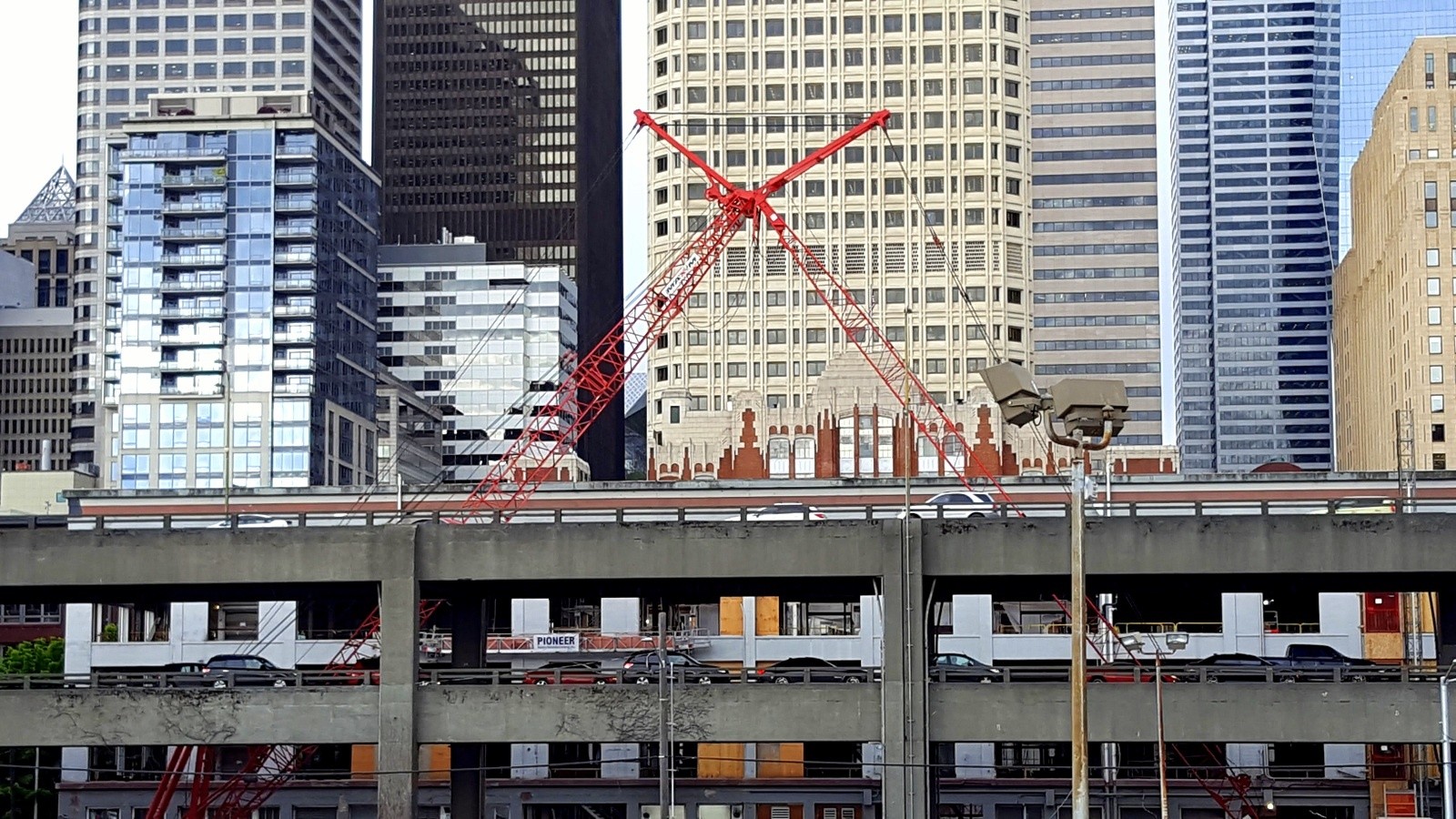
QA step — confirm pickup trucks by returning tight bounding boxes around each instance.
[1256,643,1381,684]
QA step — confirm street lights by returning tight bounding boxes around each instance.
[977,360,1134,819]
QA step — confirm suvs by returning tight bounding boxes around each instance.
[621,649,732,685]
[897,490,998,520]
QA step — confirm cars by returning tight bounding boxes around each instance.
[723,501,827,522]
[520,660,619,685]
[1178,653,1300,683]
[206,512,289,528]
[151,654,296,689]
[334,656,444,686]
[1086,661,1181,683]
[928,653,1003,684]
[1303,494,1396,515]
[756,657,868,685]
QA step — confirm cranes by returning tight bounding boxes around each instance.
[144,108,1279,819]
